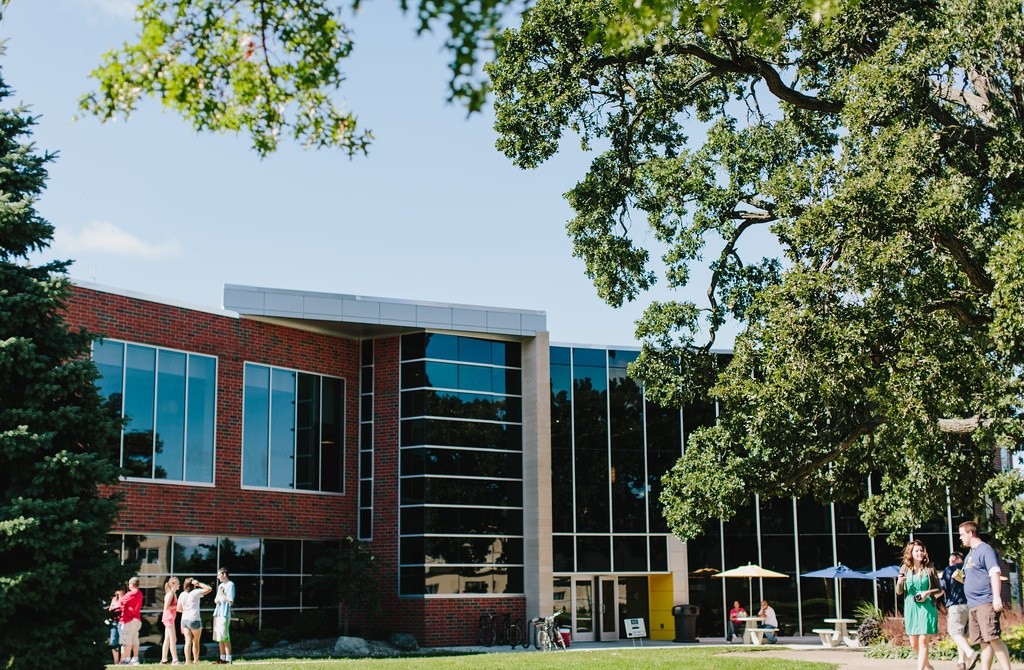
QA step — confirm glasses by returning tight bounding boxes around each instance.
[909,540,920,545]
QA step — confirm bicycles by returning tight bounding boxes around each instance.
[479,611,522,648]
[532,611,566,651]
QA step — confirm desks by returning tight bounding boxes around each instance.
[736,617,765,644]
[824,619,857,647]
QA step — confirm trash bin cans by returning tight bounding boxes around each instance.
[671,604,700,643]
[554,628,571,647]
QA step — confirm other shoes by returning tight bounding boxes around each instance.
[965,650,981,670]
[956,663,966,670]
[213,659,231,665]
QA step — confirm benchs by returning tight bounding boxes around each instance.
[812,629,839,647]
[848,630,860,647]
[748,628,780,645]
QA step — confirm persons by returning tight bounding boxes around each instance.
[896,539,942,670]
[108,567,235,664]
[934,521,1011,670]
[726,599,779,645]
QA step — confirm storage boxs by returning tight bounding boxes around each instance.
[556,629,570,647]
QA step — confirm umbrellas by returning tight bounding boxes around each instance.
[711,562,789,618]
[863,565,901,617]
[800,561,880,619]
